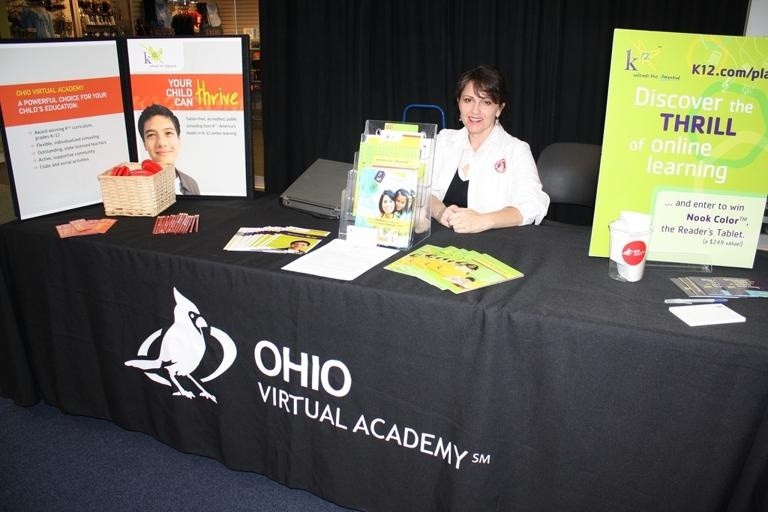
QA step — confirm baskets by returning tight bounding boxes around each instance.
[97,162,176,218]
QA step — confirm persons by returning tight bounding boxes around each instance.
[138,103,200,196]
[394,188,410,217]
[429,66,551,234]
[455,263,479,272]
[170,13,196,37]
[379,191,398,221]
[26,4,55,40]
[269,239,310,255]
[451,277,476,288]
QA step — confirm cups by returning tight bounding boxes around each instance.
[606,219,651,284]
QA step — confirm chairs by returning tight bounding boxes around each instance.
[534,142,600,226]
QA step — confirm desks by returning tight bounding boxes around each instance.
[1,191,766,512]
